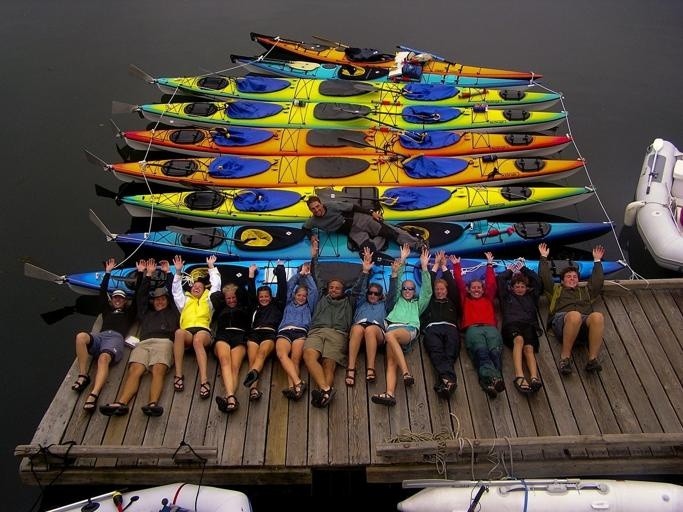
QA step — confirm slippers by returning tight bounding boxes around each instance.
[243,368,413,406]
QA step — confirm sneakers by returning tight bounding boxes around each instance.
[420,240,431,257]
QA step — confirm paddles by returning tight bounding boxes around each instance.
[111,275,166,283]
[180,88,224,102]
[311,34,352,48]
[315,188,400,206]
[337,134,411,159]
[646,138,663,194]
[146,163,234,175]
[359,249,419,269]
[166,226,256,245]
[333,106,434,140]
[179,180,235,198]
[355,84,412,95]
[402,479,578,485]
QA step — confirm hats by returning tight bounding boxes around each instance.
[112,289,127,299]
[149,285,170,298]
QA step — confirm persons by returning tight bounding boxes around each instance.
[99,258,179,416]
[303,236,372,407]
[171,252,221,398]
[538,241,607,375]
[209,284,247,413]
[345,258,402,386]
[70,256,145,412]
[370,242,432,405]
[420,249,461,398]
[495,262,544,394]
[242,262,288,400]
[306,194,427,255]
[275,262,320,401]
[449,251,508,398]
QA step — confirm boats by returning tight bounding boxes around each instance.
[127,63,565,103]
[624,138,682,275]
[24,260,630,296]
[43,482,253,511]
[110,99,568,129]
[228,52,543,92]
[396,478,682,512]
[80,147,589,192]
[88,208,616,267]
[248,31,543,80]
[107,119,576,158]
[94,182,596,228]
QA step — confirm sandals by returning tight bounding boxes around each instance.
[72,373,91,393]
[83,392,101,412]
[99,401,129,417]
[215,395,240,413]
[141,401,164,417]
[431,354,603,399]
[199,382,210,400]
[174,375,185,392]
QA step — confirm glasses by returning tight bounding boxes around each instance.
[367,291,380,296]
[401,286,415,291]
